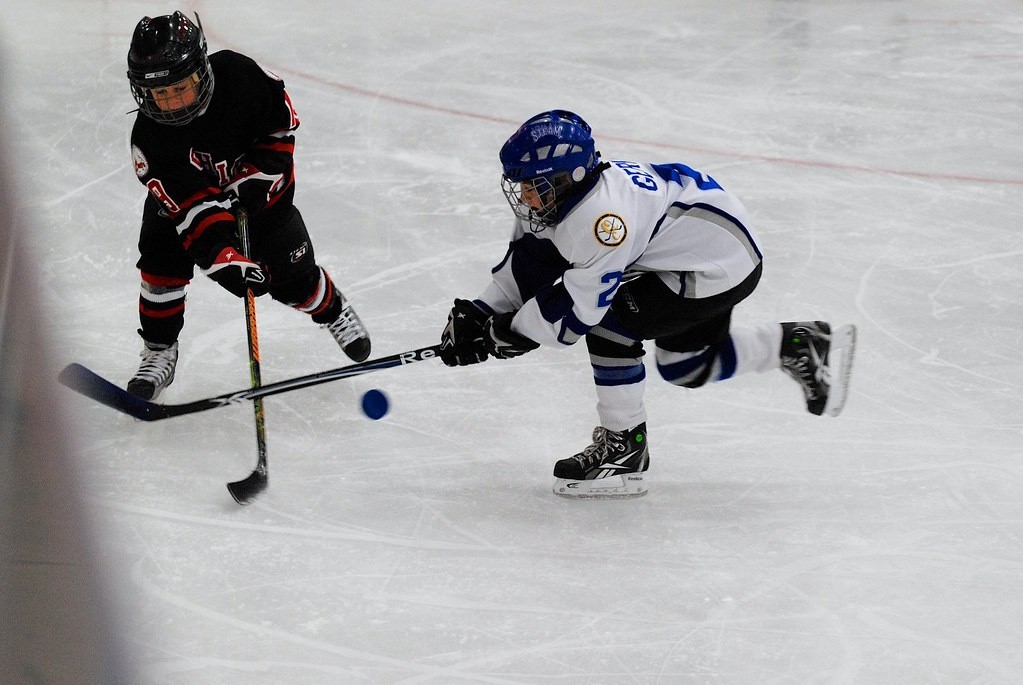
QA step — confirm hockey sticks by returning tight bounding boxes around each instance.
[56,342,442,423]
[225,206,269,506]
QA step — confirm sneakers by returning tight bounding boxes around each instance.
[776,322,857,417]
[127,328,184,400]
[553,421,650,496]
[309,265,371,362]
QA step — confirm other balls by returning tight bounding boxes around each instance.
[359,388,390,420]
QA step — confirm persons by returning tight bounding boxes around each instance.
[437,109,857,500]
[125,11,371,404]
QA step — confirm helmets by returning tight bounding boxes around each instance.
[126,11,216,127]
[497,110,603,234]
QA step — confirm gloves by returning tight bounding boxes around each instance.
[200,248,270,299]
[439,298,495,367]
[480,310,540,359]
[219,156,284,217]
